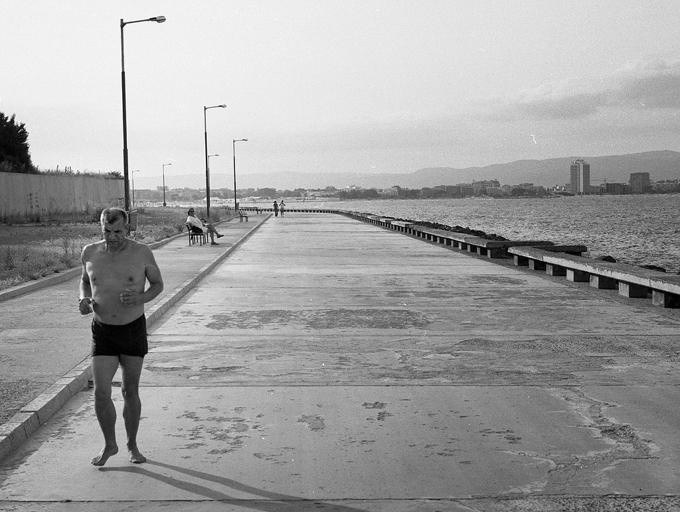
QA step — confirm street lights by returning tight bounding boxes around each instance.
[233,138,248,212]
[203,104,227,215]
[163,163,172,206]
[132,170,140,207]
[120,16,166,234]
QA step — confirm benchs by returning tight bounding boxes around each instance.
[185,218,208,246]
[239,211,248,222]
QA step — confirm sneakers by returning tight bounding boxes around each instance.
[210,234,225,245]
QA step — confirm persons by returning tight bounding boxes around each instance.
[273,201,279,217]
[279,199,286,216]
[79,207,164,467]
[186,208,224,245]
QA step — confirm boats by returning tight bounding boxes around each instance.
[204,197,262,204]
